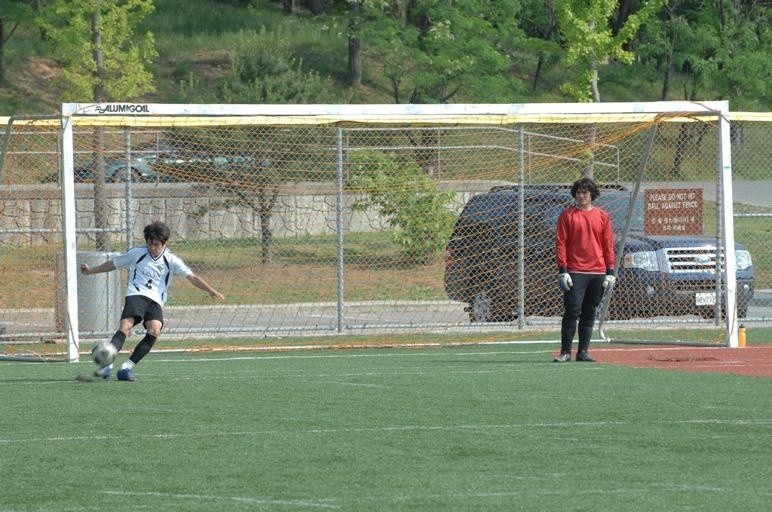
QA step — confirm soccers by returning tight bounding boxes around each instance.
[92,342,117,366]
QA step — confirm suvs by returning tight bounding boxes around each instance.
[443,181,756,324]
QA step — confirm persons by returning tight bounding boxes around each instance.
[554,178,616,362]
[81,221,224,380]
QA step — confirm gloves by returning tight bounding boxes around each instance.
[603,269,616,296]
[558,268,573,292]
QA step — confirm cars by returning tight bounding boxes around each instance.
[70,139,281,185]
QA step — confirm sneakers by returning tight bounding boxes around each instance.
[95,366,113,379]
[576,352,596,362]
[554,353,571,362]
[117,368,138,380]
